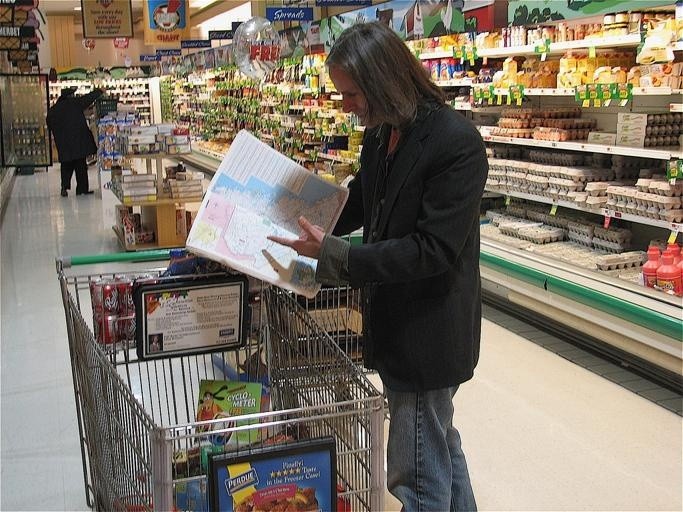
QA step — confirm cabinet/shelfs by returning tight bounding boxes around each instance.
[111,150,203,254]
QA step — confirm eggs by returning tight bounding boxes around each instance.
[485,108,683,146]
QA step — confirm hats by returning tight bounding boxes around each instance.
[61,88,76,96]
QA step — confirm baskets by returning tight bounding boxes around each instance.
[95,96,117,118]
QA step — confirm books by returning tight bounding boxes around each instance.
[184,127,350,300]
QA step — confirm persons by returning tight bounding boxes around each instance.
[46,87,107,197]
[266,19,491,512]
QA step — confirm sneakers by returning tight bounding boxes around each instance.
[61,186,68,197]
[76,187,94,194]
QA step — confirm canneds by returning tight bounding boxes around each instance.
[90,274,154,346]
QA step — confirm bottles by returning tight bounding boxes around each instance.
[500,21,601,51]
[641,242,683,296]
